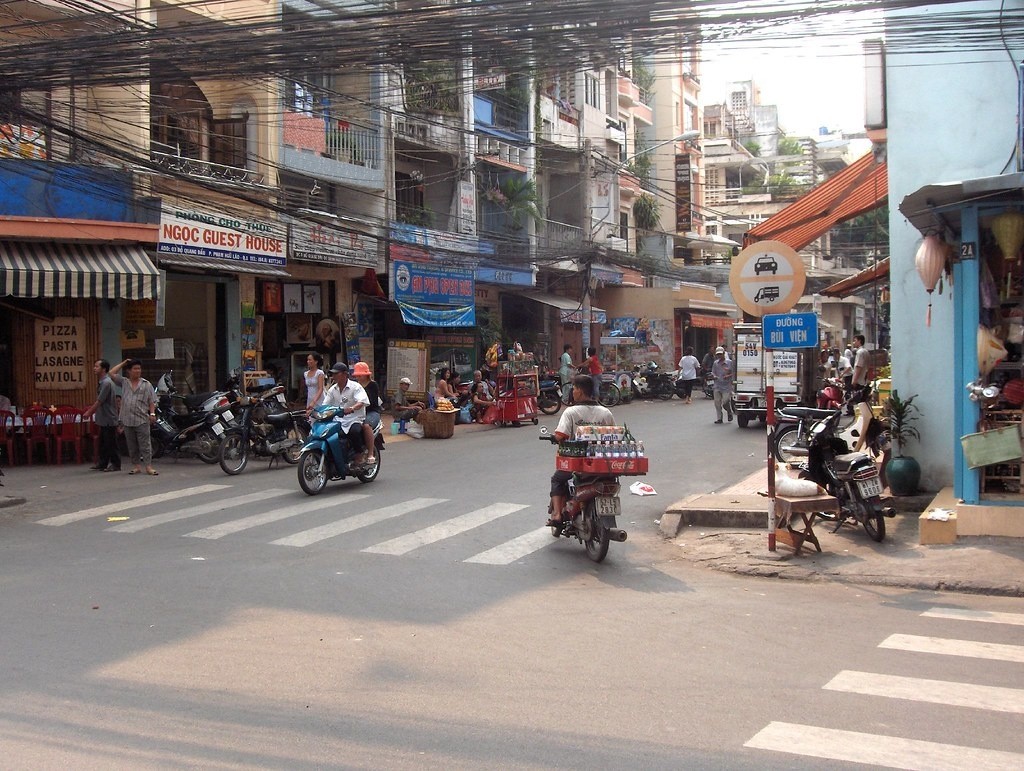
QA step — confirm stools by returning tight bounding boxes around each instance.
[394,419,410,434]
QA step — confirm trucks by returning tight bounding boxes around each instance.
[730,322,826,427]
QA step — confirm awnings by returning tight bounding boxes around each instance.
[0,240,161,300]
[510,290,606,324]
[819,255,889,300]
[742,149,887,255]
[148,250,292,277]
[686,312,735,330]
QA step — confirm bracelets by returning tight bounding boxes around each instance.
[150,413,156,416]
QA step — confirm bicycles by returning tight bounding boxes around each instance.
[559,366,621,407]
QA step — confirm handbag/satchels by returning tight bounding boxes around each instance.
[482,405,499,423]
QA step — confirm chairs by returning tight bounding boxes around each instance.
[53,406,86,465]
[21,408,54,467]
[82,404,101,465]
[0,409,19,468]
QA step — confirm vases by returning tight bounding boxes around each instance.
[417,183,425,192]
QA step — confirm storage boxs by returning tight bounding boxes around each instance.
[555,456,582,472]
[581,456,649,474]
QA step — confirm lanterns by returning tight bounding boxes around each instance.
[916,234,953,328]
[992,211,1024,269]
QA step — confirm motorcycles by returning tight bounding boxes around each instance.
[804,410,896,541]
[218,395,314,475]
[538,424,628,563]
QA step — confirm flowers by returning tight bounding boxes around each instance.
[410,170,424,185]
[500,197,511,206]
[487,187,503,202]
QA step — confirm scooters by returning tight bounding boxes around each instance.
[143,367,288,465]
[298,396,386,496]
[814,364,843,411]
[699,367,715,399]
[527,379,564,415]
[772,381,885,470]
[598,363,676,405]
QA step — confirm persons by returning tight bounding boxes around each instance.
[390,378,426,421]
[322,362,381,468]
[546,376,618,526]
[818,334,873,416]
[559,344,575,404]
[677,346,701,403]
[82,359,122,471]
[435,368,497,423]
[574,346,603,403]
[711,347,733,424]
[303,354,328,437]
[115,395,129,456]
[109,358,160,476]
[702,345,732,372]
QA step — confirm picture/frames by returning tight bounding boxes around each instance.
[290,351,318,389]
[282,282,303,313]
[302,284,323,314]
[285,314,313,345]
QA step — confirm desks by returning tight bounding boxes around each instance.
[757,489,841,557]
[0,413,95,464]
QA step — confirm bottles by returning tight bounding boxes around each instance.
[637,441,645,458]
[10,406,16,417]
[629,440,636,458]
[391,417,415,436]
[557,427,631,456]
[32,399,57,412]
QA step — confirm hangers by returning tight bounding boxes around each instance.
[311,180,321,195]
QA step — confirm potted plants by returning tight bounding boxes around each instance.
[878,389,925,497]
[326,130,360,164]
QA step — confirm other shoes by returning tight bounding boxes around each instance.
[688,399,692,401]
[354,454,365,463]
[715,419,723,423]
[104,465,121,472]
[88,466,104,471]
[728,415,733,421]
[686,401,691,404]
[477,418,483,423]
[546,518,562,526]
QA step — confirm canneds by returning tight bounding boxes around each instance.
[576,426,624,439]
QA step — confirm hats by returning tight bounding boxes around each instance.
[352,362,372,376]
[328,362,348,373]
[400,378,413,384]
[715,347,724,354]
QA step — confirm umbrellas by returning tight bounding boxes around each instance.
[687,233,741,250]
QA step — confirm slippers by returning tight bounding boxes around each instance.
[366,457,375,464]
[129,468,142,474]
[145,469,159,475]
